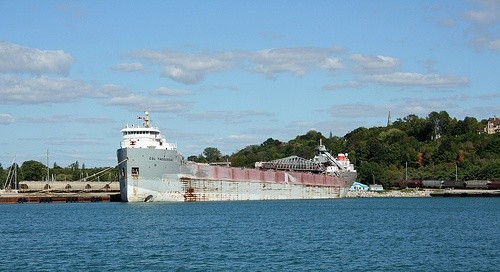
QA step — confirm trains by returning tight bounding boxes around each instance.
[408,179,500,190]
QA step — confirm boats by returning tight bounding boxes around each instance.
[430,187,500,197]
[115,106,356,202]
